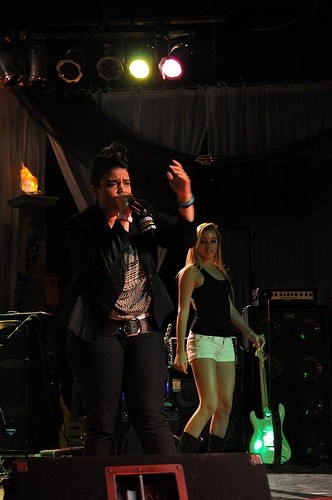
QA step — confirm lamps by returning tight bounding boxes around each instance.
[157,42,195,81]
[56,48,89,83]
[127,44,158,80]
[27,45,52,89]
[0,39,27,89]
[96,47,125,82]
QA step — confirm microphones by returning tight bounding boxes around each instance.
[127,199,147,216]
[7,315,32,341]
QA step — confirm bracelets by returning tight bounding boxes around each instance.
[248,331,255,341]
[177,196,195,208]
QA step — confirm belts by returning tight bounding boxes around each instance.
[97,316,153,337]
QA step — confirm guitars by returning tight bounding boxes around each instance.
[59,375,90,455]
[248,347,292,465]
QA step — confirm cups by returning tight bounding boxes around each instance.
[46,277,58,305]
[16,459,27,473]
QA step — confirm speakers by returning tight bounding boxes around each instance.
[242,305,332,474]
[2,453,272,500]
[0,312,60,453]
[171,374,254,454]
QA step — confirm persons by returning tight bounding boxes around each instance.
[174,222,265,452]
[63,142,195,457]
